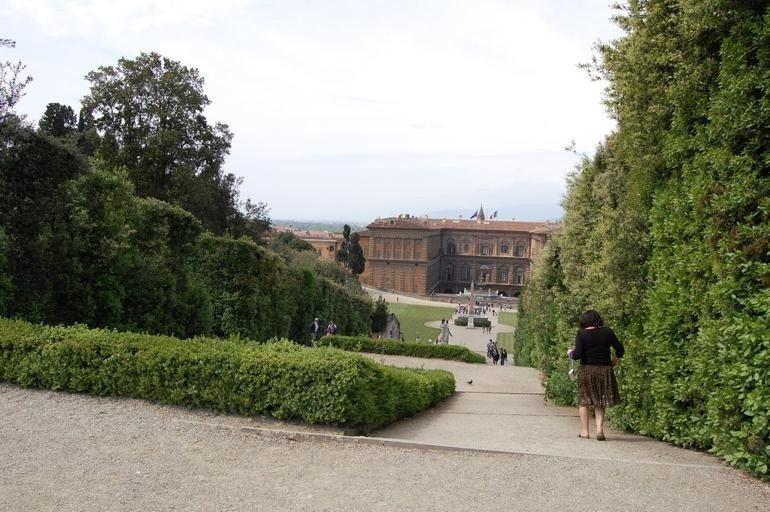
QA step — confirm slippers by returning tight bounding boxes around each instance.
[596,433,606,440]
[578,433,590,439]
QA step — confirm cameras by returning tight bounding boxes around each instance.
[569,369,576,379]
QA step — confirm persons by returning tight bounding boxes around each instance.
[567,310,625,440]
[415,297,512,366]
[326,320,337,336]
[309,318,320,339]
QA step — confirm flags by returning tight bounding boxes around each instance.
[470,211,477,220]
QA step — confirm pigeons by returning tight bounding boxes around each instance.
[467,380,472,384]
